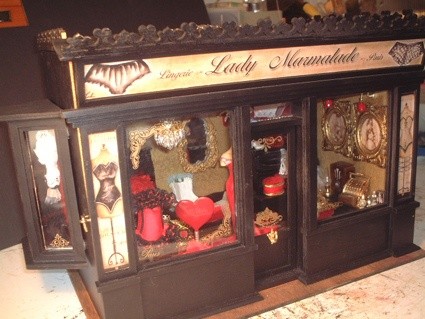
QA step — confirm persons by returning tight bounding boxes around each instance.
[92,147,124,219]
[219,146,235,228]
